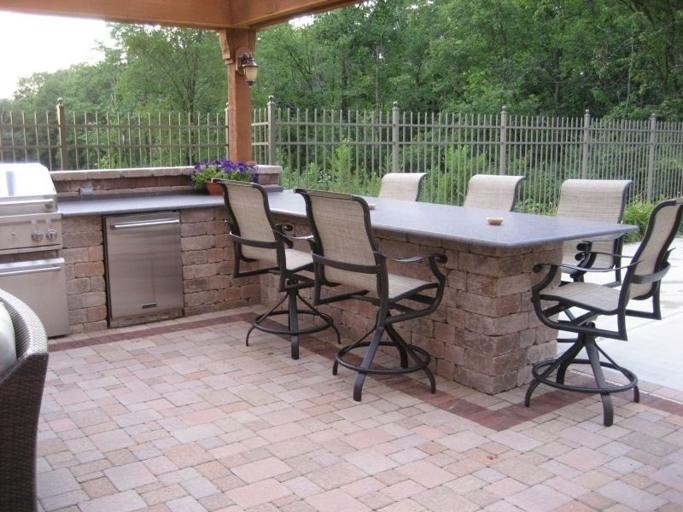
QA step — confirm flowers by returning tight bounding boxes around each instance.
[190,159,259,193]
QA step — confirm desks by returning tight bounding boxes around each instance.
[251,180,640,398]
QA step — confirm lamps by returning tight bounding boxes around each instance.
[240,51,260,87]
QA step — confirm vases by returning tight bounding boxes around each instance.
[205,179,225,196]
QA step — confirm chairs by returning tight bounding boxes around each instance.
[292,185,449,403]
[521,195,682,428]
[376,172,430,202]
[550,176,638,322]
[460,173,527,211]
[214,176,342,362]
